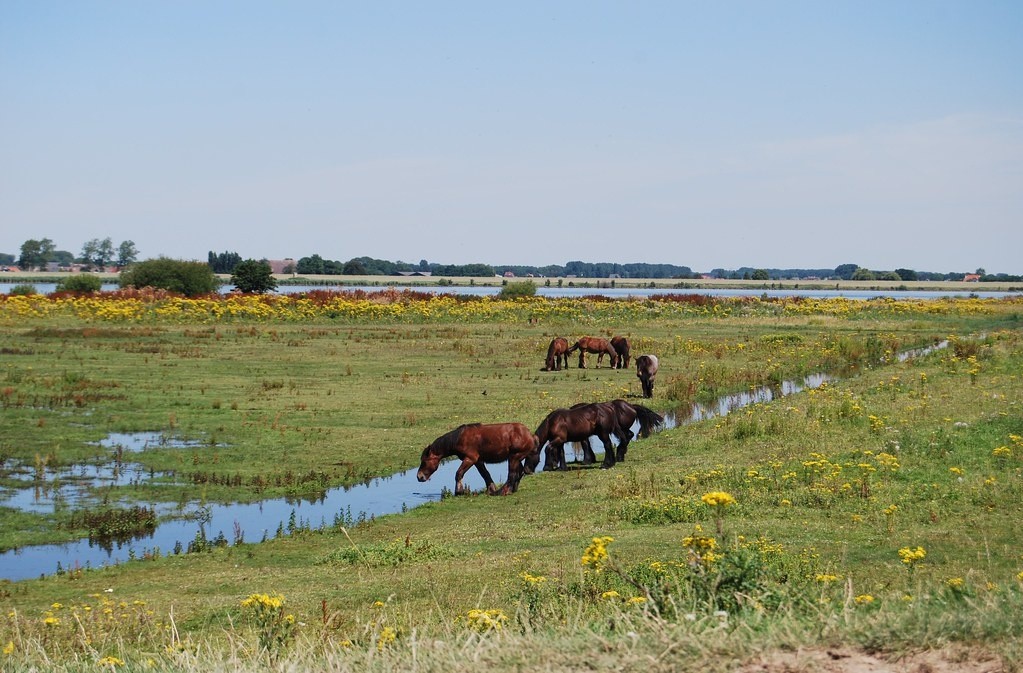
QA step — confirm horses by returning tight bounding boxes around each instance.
[544,335,658,399]
[416,399,665,496]
